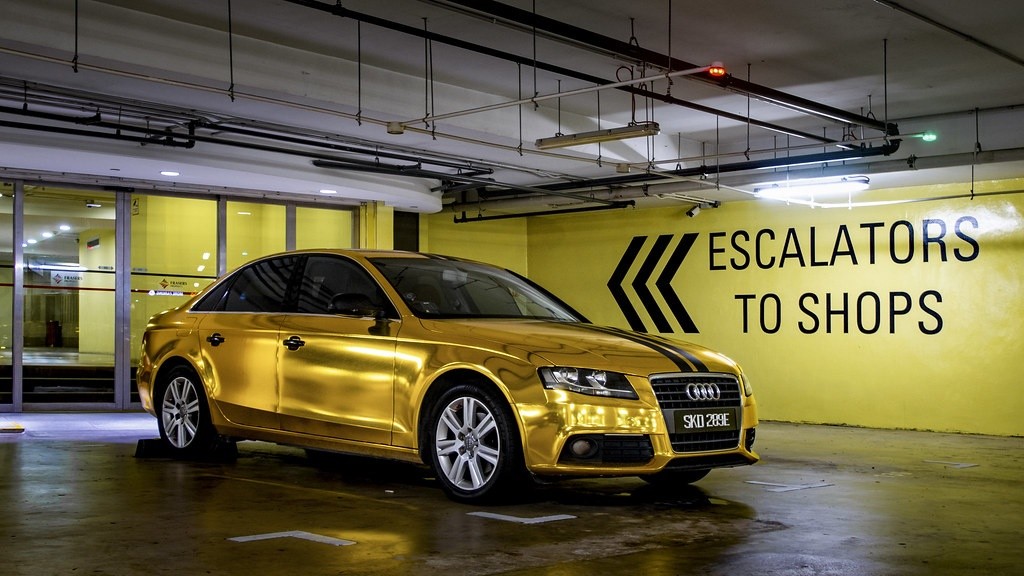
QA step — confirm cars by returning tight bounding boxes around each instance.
[136,246,760,506]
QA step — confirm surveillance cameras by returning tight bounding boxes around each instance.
[686,206,701,218]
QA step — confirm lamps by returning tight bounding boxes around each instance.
[535,79,661,150]
